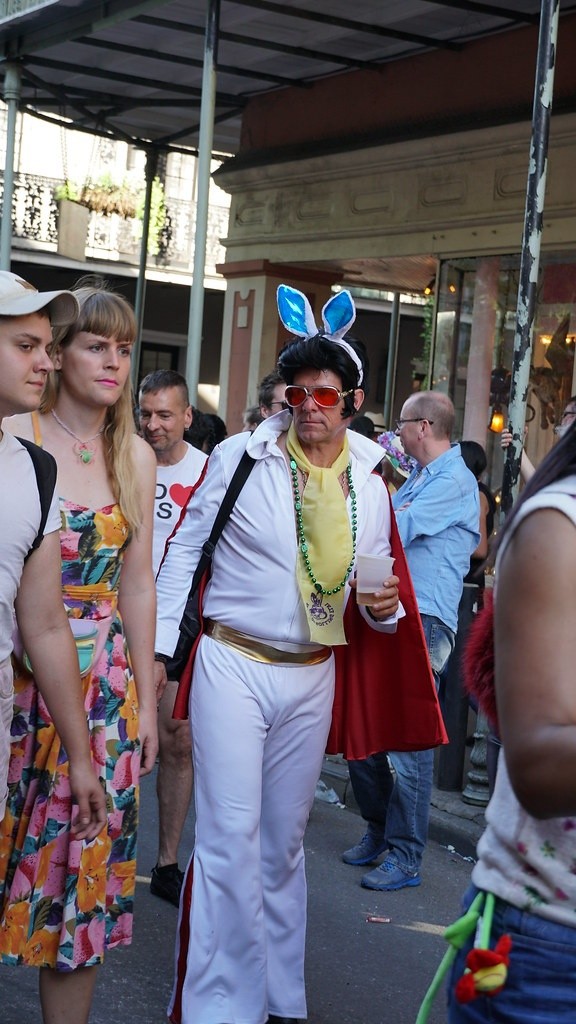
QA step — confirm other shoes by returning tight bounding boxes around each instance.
[150,861,185,907]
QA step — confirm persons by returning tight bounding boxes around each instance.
[139,369,209,906]
[156,285,450,1024]
[438,418,576,1024]
[342,390,482,891]
[0,269,107,842]
[0,286,160,1024]
[501,395,576,485]
[458,440,496,588]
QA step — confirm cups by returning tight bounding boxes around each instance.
[356,554,396,606]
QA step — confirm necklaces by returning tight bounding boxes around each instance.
[51,407,105,465]
[290,455,357,595]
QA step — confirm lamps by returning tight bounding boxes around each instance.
[487,369,512,434]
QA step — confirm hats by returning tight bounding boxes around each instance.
[0,271,80,327]
[378,431,415,478]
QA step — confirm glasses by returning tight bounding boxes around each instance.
[283,385,356,408]
[395,418,433,430]
[267,400,293,414]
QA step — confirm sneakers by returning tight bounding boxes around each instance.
[361,860,421,890]
[342,832,389,864]
